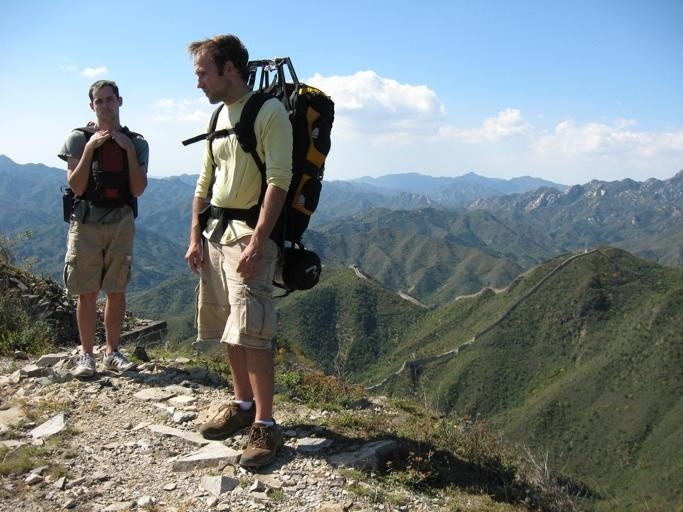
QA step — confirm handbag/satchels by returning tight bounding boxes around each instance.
[275,241,321,294]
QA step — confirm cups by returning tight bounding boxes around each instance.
[60,185,74,223]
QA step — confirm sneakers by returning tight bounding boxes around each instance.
[71,352,97,377]
[102,350,136,373]
[198,396,256,439]
[238,418,282,467]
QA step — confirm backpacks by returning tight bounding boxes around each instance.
[205,56,334,243]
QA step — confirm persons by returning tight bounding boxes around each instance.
[55,80,149,380]
[181,33,293,468]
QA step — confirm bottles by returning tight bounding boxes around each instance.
[297,194,305,207]
